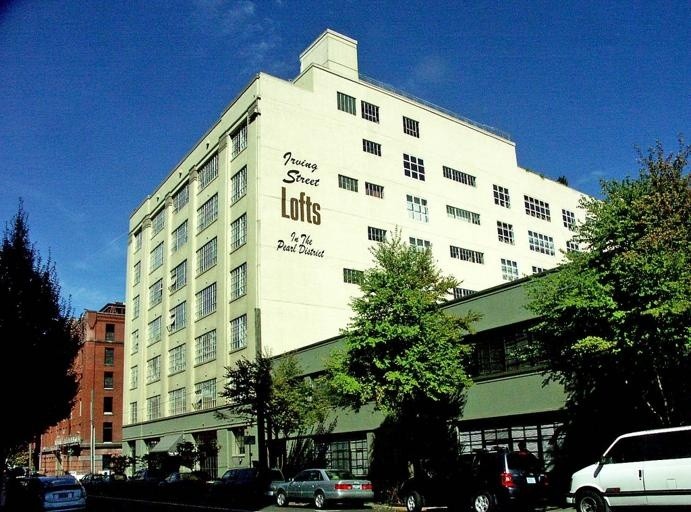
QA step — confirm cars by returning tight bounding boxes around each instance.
[398,452,547,512]
[16,468,285,512]
[277,468,374,508]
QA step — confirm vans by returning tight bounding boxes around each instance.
[567,424,691,511]
